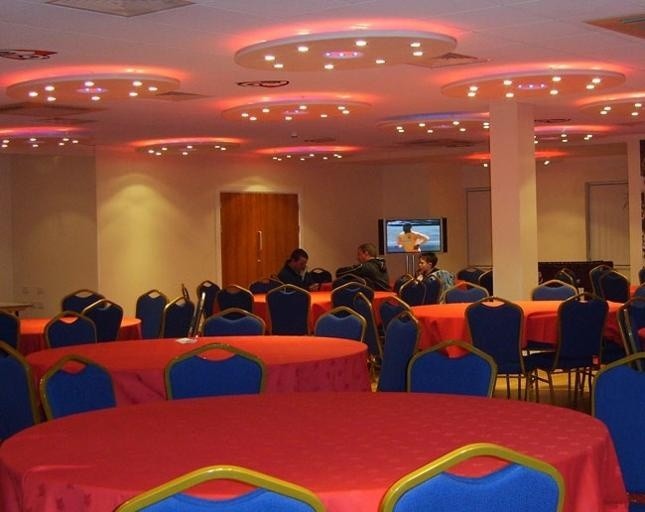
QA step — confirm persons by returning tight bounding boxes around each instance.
[397,223,428,252]
[280,249,320,292]
[336,243,390,291]
[414,252,440,281]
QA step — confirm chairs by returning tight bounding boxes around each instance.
[1,255,645,512]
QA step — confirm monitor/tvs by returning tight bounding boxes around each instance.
[378,216,447,255]
